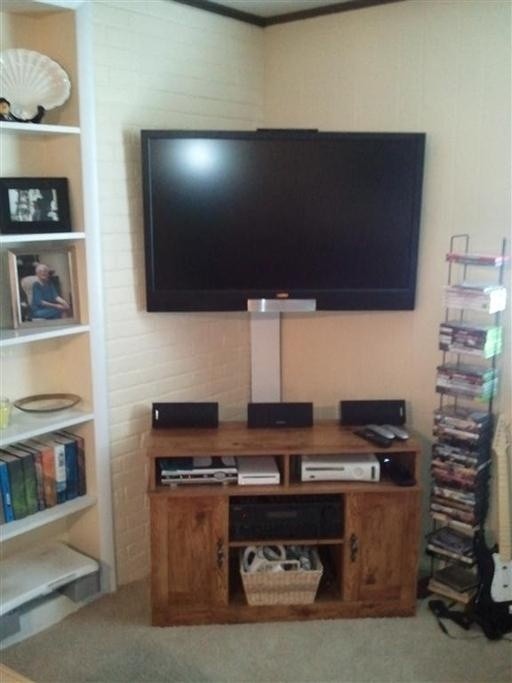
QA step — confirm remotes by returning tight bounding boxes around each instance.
[367,424,395,440]
[353,428,392,448]
[385,425,409,440]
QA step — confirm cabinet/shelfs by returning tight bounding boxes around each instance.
[140,422,422,626]
[0,0,120,653]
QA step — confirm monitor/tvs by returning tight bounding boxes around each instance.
[141,128,426,311]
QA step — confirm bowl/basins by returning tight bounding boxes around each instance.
[11,392,82,421]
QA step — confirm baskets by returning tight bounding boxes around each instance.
[239,548,325,607]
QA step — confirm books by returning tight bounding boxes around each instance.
[427,249,507,603]
[0,429,88,526]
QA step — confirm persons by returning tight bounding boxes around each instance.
[27,264,70,320]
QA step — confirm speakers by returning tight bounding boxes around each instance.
[340,400,405,427]
[248,402,313,428]
[153,402,219,429]
